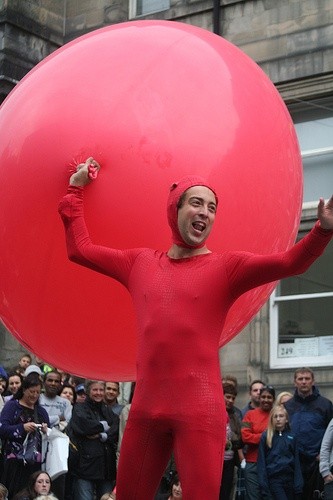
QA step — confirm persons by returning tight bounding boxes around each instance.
[1,355,333,500]
[58,158,333,500]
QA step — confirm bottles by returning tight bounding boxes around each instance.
[226,422,231,442]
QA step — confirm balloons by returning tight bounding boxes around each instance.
[0,20,302,379]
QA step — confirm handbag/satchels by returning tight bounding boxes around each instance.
[38,428,70,480]
[7,429,42,466]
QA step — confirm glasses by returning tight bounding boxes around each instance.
[259,386,275,394]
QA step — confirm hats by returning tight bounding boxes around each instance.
[75,384,85,392]
[25,364,41,376]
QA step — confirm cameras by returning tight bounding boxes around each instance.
[36,424,42,428]
